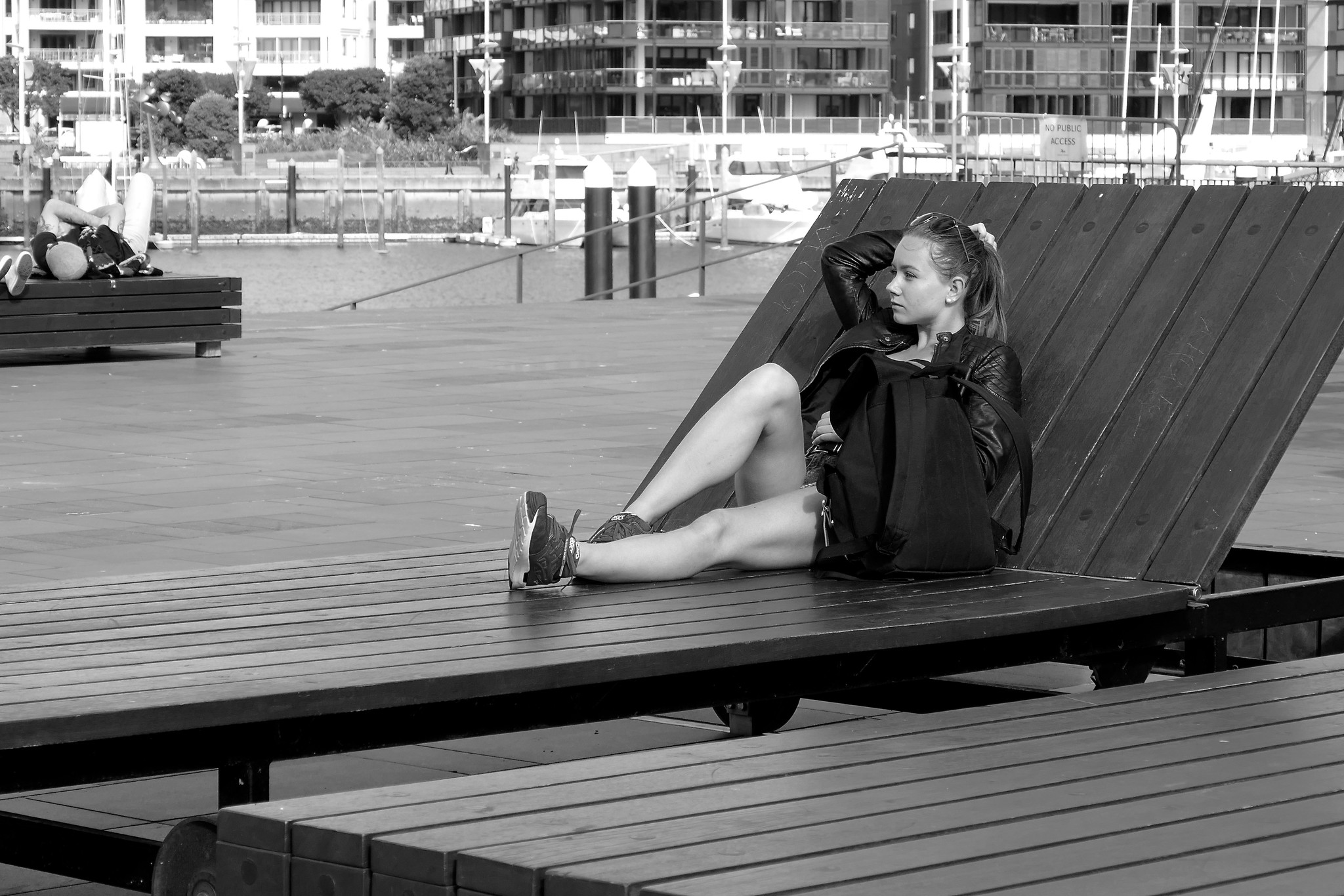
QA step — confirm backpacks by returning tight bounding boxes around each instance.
[808,352,1033,578]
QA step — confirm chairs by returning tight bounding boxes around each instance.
[0,176,1344,896]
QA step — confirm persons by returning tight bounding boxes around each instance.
[0,169,163,297]
[507,211,1022,590]
[443,147,454,175]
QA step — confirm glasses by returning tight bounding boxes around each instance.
[910,212,970,262]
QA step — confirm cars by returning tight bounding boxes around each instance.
[35,126,75,151]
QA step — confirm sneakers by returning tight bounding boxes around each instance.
[507,493,581,589]
[0,251,33,297]
[586,510,653,543]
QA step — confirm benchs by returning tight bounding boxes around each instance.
[0,267,242,361]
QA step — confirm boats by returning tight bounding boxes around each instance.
[840,122,969,187]
[492,152,633,246]
[698,151,823,243]
